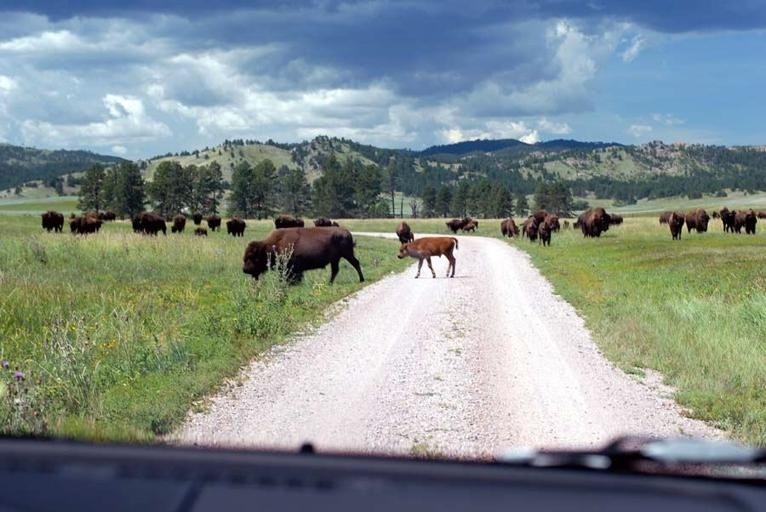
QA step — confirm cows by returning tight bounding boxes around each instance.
[396,222,413,244]
[446,217,479,234]
[129,211,222,236]
[660,207,766,241]
[226,220,246,237]
[500,207,623,246]
[243,214,364,287]
[41,211,115,236]
[398,237,458,278]
